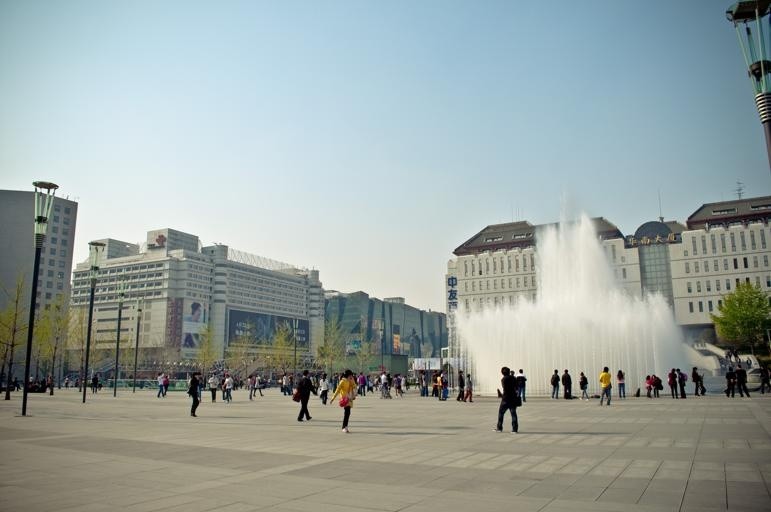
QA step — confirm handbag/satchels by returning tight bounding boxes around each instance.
[338,396,348,407]
[292,391,300,402]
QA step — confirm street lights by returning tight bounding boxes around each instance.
[113,293,127,397]
[132,309,143,393]
[244,358,248,386]
[82,241,106,403]
[291,318,298,378]
[727,1,771,174]
[378,328,384,373]
[21,180,60,417]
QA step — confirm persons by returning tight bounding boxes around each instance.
[759,365,770,394]
[65,374,98,393]
[726,364,750,398]
[157,372,170,398]
[183,301,202,347]
[669,368,686,398]
[646,375,661,398]
[517,370,527,402]
[616,370,625,398]
[562,369,572,399]
[457,371,473,402]
[294,369,318,422]
[208,371,293,403]
[552,370,560,399]
[493,367,522,435]
[692,367,700,396]
[400,327,421,358]
[375,369,449,401]
[308,372,374,406]
[579,372,589,400]
[12,374,55,396]
[186,373,199,417]
[600,367,612,405]
[330,370,357,433]
